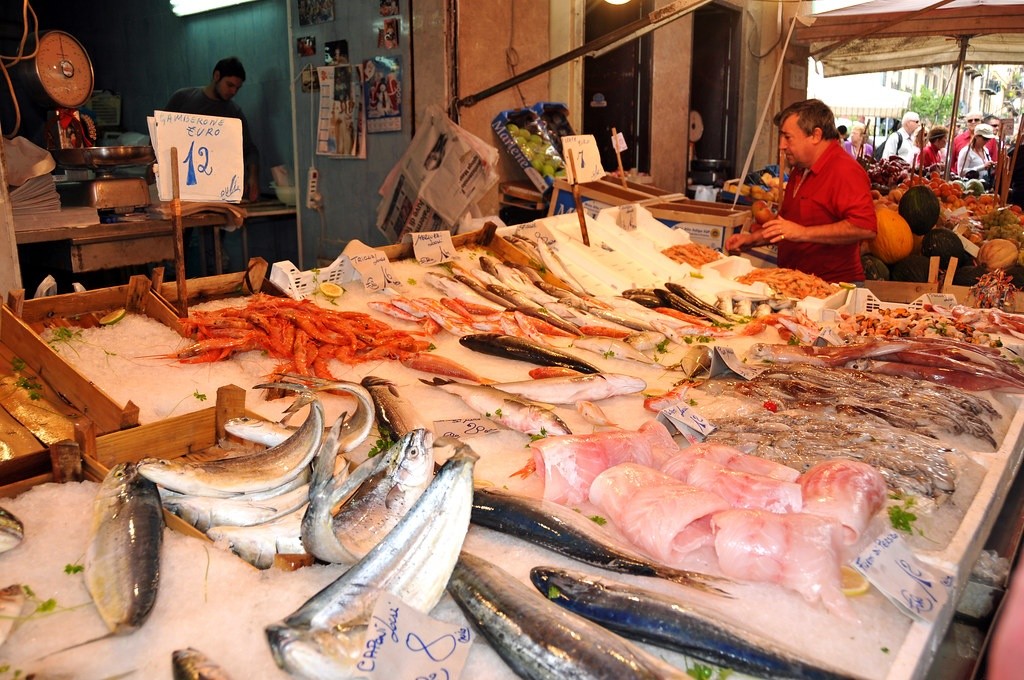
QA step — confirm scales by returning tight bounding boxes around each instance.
[687,106,728,200]
[31,29,156,218]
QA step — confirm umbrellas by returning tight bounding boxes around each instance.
[792,0,1024,185]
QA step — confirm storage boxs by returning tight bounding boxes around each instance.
[531,101,577,158]
[721,164,789,206]
[491,107,568,197]
[547,177,657,218]
[645,202,752,257]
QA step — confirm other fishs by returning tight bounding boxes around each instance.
[364,219,1023,519]
[0,373,891,680]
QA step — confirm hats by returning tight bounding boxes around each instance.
[974,123,995,138]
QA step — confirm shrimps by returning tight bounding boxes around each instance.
[149,274,481,396]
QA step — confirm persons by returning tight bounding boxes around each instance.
[297,1,402,164]
[160,57,260,201]
[724,99,877,286]
[837,112,1024,209]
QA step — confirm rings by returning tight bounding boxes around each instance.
[779,229,782,234]
[781,235,784,239]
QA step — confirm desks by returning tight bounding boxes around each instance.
[198,206,295,278]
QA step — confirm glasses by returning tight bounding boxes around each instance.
[968,118,979,123]
[991,124,999,127]
[916,121,919,123]
